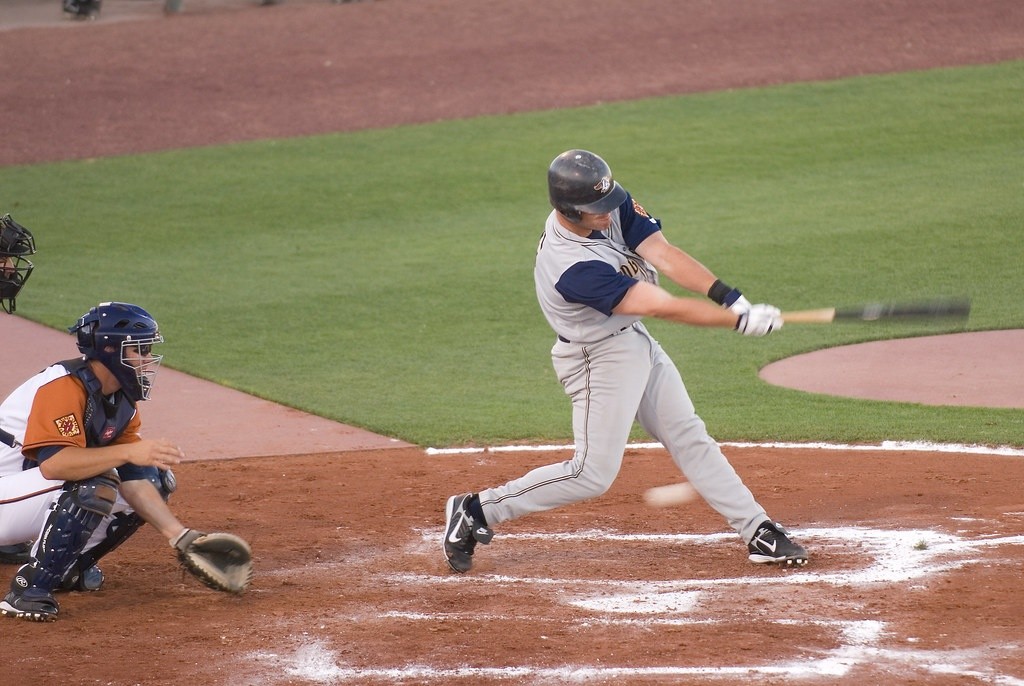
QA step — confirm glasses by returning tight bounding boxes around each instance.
[130,346,152,356]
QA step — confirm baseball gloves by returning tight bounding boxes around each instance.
[176,526,252,597]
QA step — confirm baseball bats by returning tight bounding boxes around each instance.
[777,297,974,330]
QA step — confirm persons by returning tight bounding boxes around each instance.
[442,150,808,573]
[0,301,253,623]
[0,213,37,314]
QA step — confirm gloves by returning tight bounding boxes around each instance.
[720,287,784,337]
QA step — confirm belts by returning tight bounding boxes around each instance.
[558,326,626,343]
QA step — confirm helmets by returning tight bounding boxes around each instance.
[548,149,627,223]
[0,217,24,300]
[68,302,158,402]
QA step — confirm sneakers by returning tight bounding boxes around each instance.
[71,561,104,591]
[748,519,808,566]
[443,493,494,574]
[0,589,58,622]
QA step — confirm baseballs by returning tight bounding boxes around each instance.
[646,483,693,511]
